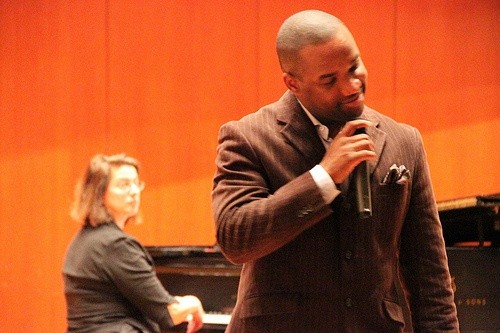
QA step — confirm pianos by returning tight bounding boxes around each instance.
[139,191,500,333]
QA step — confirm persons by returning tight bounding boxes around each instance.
[62,152,205,333]
[211,8,461,333]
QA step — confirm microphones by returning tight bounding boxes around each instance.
[349,117,372,219]
